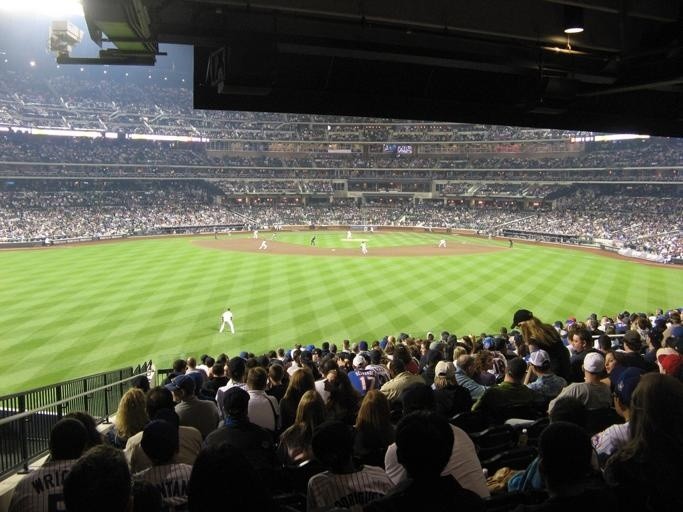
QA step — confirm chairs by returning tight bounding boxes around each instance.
[369,408,625,512]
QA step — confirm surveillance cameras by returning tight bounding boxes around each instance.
[49,18,86,45]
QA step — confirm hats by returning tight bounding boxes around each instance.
[353,341,380,368]
[511,310,533,328]
[288,345,315,359]
[530,350,550,366]
[583,353,604,372]
[435,360,455,377]
[483,337,494,348]
[656,347,683,374]
[615,368,639,396]
[163,372,202,394]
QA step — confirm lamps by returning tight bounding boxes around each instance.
[564,6,584,33]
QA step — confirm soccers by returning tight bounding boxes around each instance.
[332,249,334,251]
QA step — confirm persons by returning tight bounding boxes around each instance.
[5,307,683,512]
[1,72,683,281]
